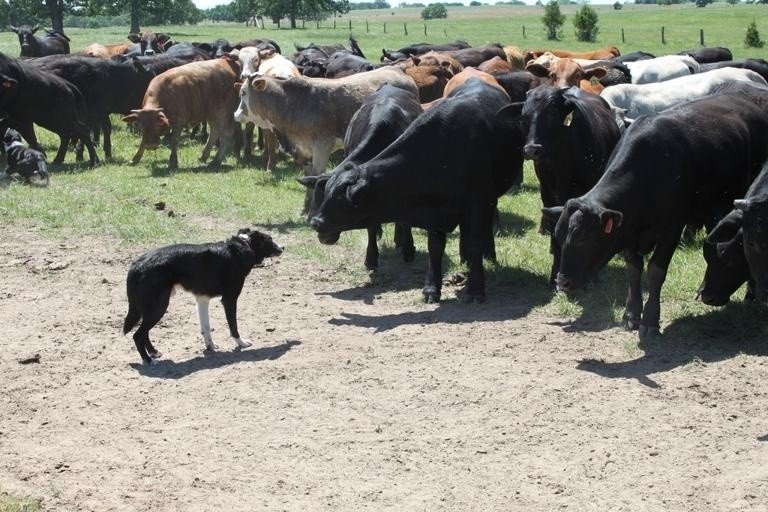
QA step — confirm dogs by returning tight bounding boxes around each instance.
[3,122,56,190]
[121,225,287,370]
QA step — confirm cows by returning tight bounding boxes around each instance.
[699,59,768,80]
[233,65,421,217]
[536,47,625,61]
[623,55,702,84]
[298,84,420,270]
[541,84,768,330]
[122,56,238,172]
[231,45,271,166]
[317,79,524,303]
[22,54,118,164]
[525,58,609,91]
[7,25,73,59]
[0,50,102,171]
[613,50,658,61]
[259,53,304,174]
[122,29,169,58]
[381,37,537,101]
[170,37,281,56]
[734,169,766,289]
[699,163,768,308]
[495,85,625,305]
[678,45,734,62]
[294,42,380,76]
[599,66,768,133]
